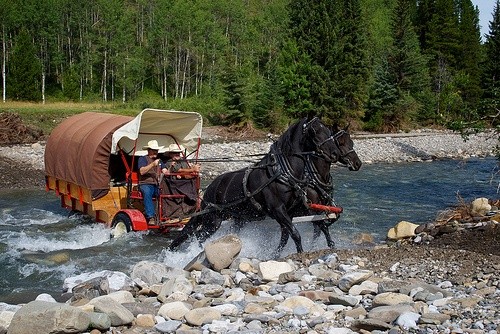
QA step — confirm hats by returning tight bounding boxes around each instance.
[163,144,183,157]
[141,140,164,150]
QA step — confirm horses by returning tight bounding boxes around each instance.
[234,120,362,251]
[169,109,340,257]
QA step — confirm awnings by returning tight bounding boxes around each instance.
[110,108,203,174]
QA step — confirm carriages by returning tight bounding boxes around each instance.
[43,108,363,263]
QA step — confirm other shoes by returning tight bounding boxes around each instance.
[148,218,155,225]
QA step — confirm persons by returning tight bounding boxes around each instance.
[160,144,201,173]
[138,140,176,225]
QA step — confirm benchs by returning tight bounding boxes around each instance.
[124,170,157,200]
